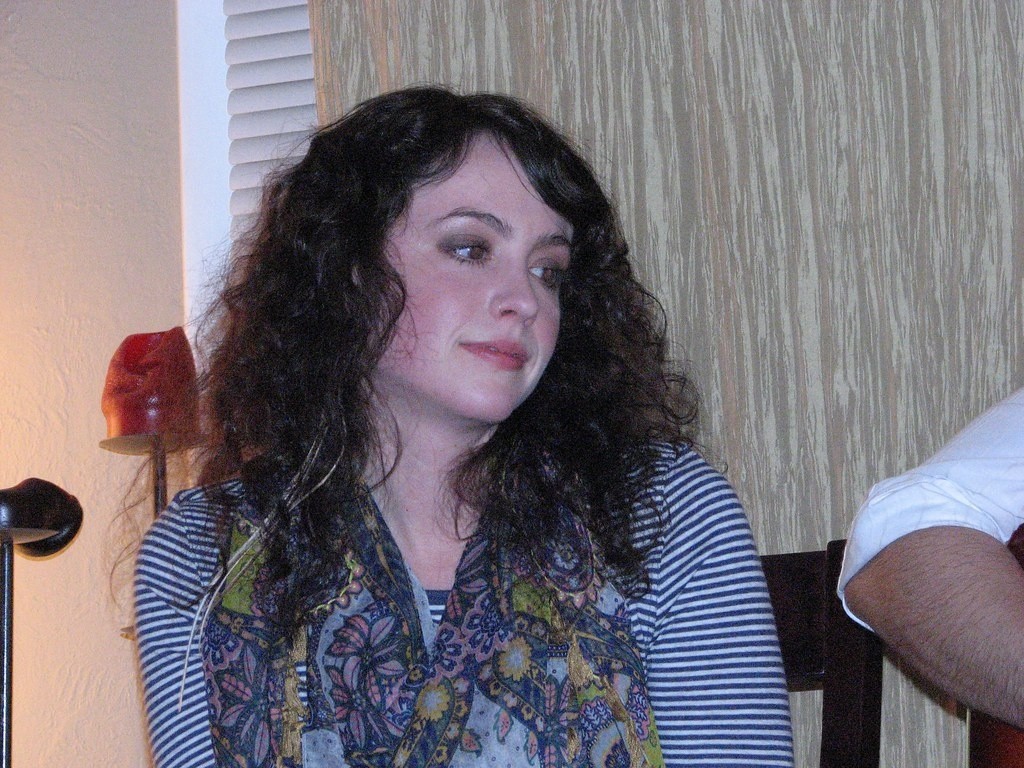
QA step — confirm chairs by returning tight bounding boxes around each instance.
[757,540,882,768]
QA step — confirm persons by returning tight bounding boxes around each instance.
[132,85,792,768]
[840,387,1024,726]
